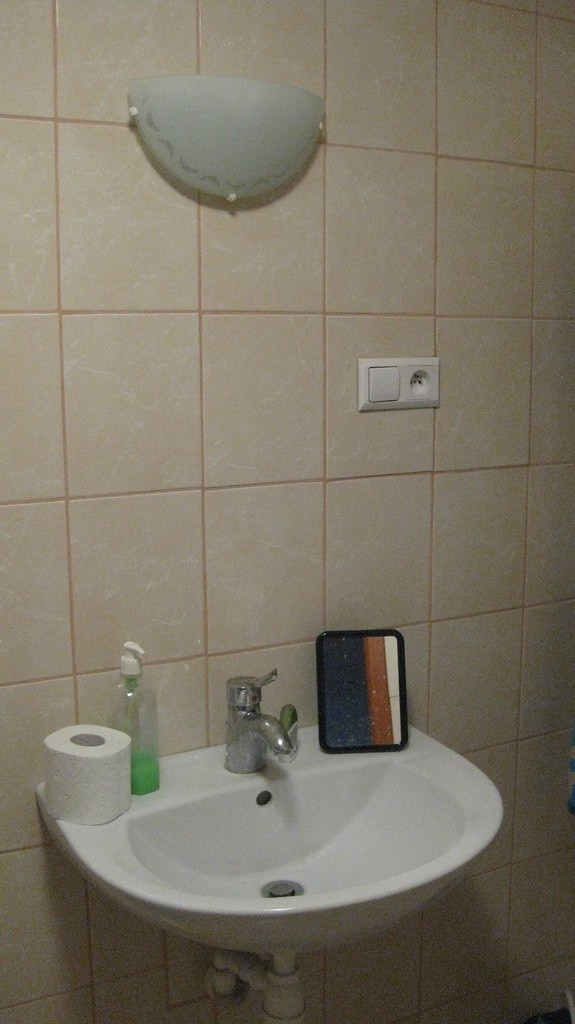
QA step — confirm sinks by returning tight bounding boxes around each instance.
[32,723,505,955]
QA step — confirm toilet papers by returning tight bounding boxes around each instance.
[41,722,133,826]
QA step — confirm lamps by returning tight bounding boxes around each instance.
[127,74,327,204]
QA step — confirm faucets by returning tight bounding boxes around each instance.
[222,666,294,775]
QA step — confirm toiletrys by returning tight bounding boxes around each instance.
[278,704,299,763]
[107,640,161,797]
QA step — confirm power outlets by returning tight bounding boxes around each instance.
[357,356,440,414]
